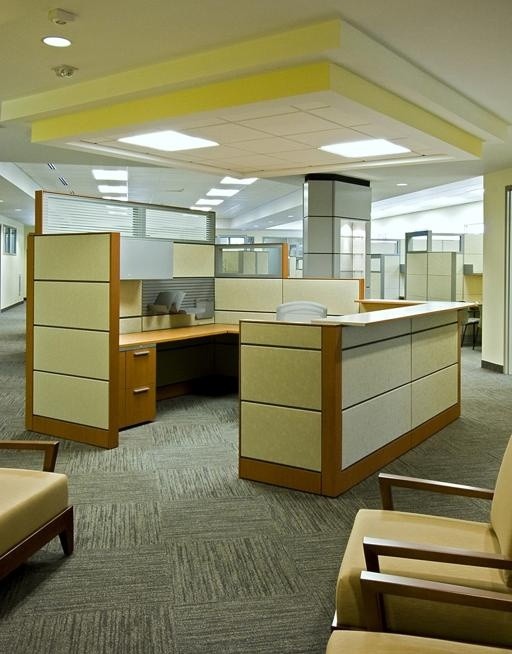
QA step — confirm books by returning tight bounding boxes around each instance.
[149,290,186,313]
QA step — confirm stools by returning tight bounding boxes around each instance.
[0,436,76,578]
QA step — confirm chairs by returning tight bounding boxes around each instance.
[325,571,511,653]
[461,305,482,350]
[331,426,512,630]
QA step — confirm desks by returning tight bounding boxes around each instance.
[117,323,241,432]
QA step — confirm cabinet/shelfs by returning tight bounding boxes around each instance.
[117,344,157,432]
